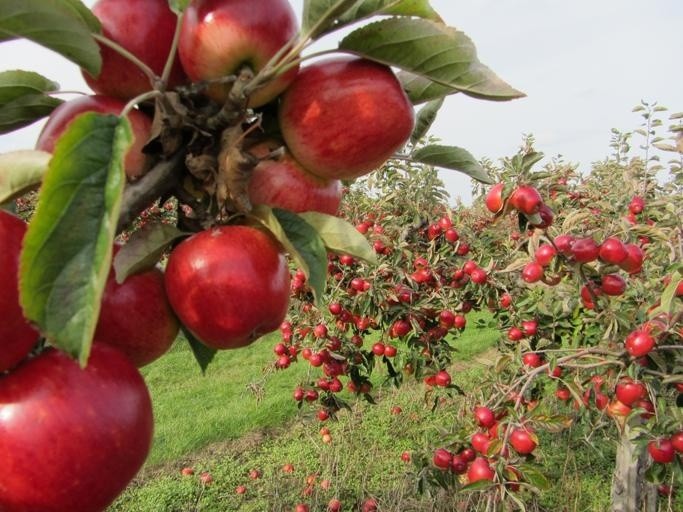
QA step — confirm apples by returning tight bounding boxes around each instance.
[0,0,683,512]
